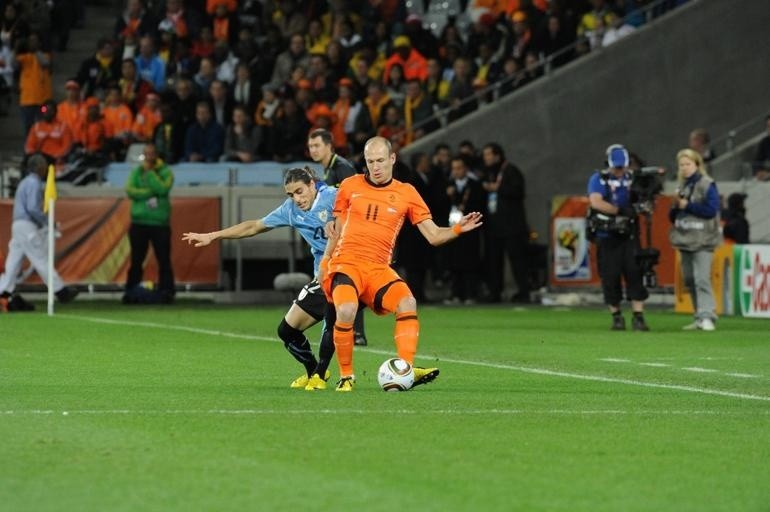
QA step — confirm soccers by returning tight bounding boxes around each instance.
[379,359,414,394]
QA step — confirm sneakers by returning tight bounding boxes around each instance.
[336,376,355,392]
[305,373,327,391]
[412,366,439,387]
[290,369,329,387]
[354,332,366,345]
[612,316,715,331]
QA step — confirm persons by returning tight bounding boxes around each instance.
[690,129,715,163]
[754,115,769,182]
[317,137,483,392]
[0,0,662,305]
[658,148,722,330]
[182,167,342,391]
[308,128,367,346]
[586,143,662,331]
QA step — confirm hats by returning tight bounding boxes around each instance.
[607,144,630,168]
